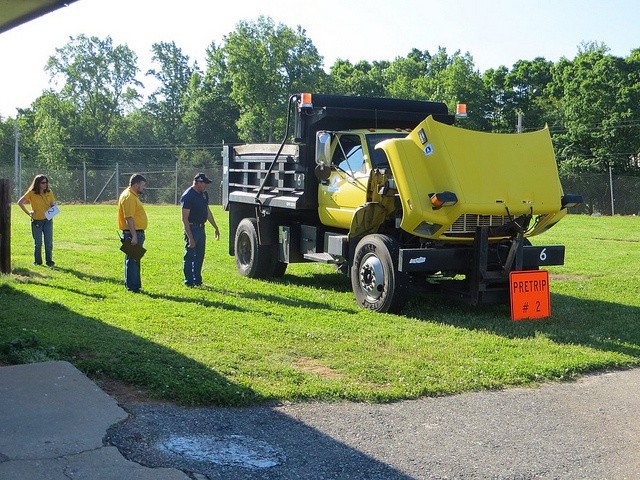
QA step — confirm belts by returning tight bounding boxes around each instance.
[189,223,205,227]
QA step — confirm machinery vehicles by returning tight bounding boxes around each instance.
[221,93,585,314]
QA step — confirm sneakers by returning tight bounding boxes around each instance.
[195,281,202,287]
[46,262,55,266]
[186,283,194,288]
[34,261,42,265]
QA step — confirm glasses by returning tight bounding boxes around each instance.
[40,182,47,184]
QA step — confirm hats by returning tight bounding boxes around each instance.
[194,173,212,183]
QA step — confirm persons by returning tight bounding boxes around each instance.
[117,174,148,294]
[17,174,56,267]
[180,173,220,288]
